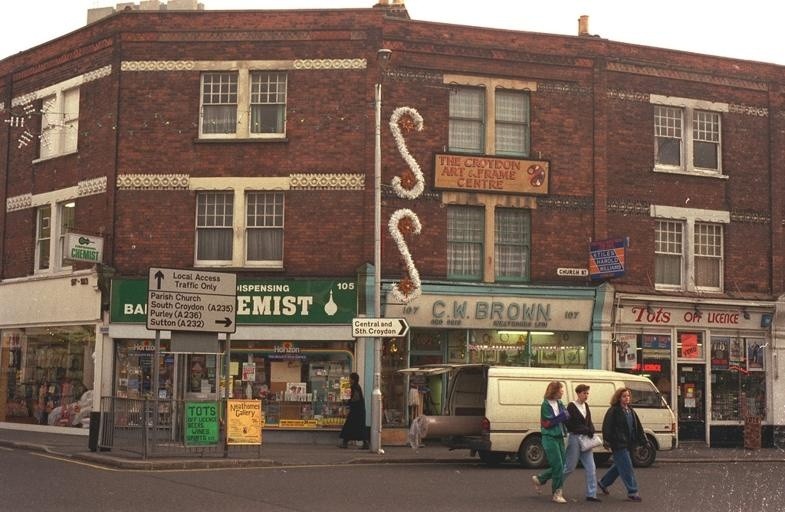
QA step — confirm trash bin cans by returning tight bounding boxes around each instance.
[744,416,761,451]
[89,410,115,453]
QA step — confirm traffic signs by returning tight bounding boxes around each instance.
[351,319,407,339]
[146,267,235,334]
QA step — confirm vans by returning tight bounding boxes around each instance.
[395,359,678,469]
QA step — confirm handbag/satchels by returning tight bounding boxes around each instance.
[578,434,602,451]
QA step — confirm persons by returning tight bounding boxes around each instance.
[750,340,761,363]
[596,386,652,501]
[562,383,602,502]
[336,372,371,448]
[531,381,573,503]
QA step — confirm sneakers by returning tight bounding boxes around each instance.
[551,488,569,503]
[360,446,370,450]
[597,481,610,495]
[338,446,347,448]
[628,494,641,502]
[533,476,543,491]
